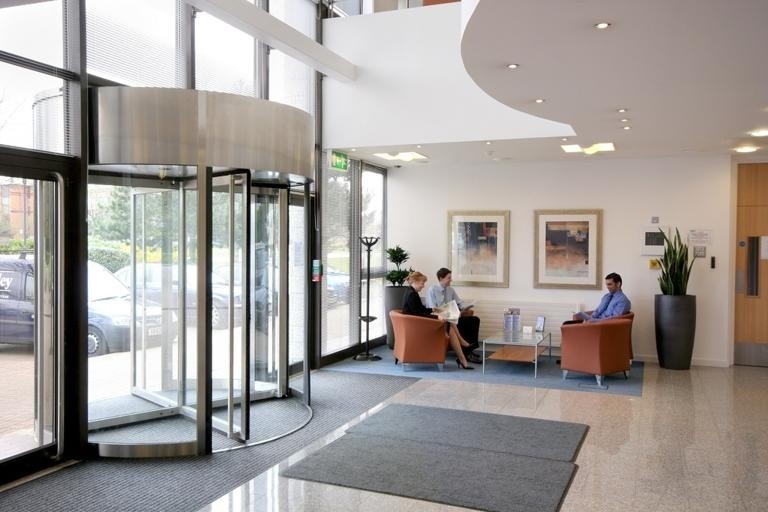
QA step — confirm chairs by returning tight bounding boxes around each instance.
[573,310,635,365]
[448,310,473,350]
[560,318,633,390]
[389,309,450,371]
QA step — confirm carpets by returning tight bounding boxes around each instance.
[345,403,590,462]
[321,344,645,396]
[278,432,591,512]
[0,369,423,512]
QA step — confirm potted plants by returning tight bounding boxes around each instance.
[383,245,415,349]
[654,228,696,370]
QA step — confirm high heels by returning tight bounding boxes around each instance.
[456,358,475,370]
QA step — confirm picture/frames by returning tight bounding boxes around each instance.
[533,209,604,291]
[447,209,510,288]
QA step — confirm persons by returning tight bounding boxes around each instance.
[426,268,482,365]
[556,273,631,365]
[402,271,476,370]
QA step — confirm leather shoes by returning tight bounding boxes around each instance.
[467,352,483,364]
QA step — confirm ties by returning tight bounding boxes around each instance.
[442,287,448,305]
[597,295,615,318]
